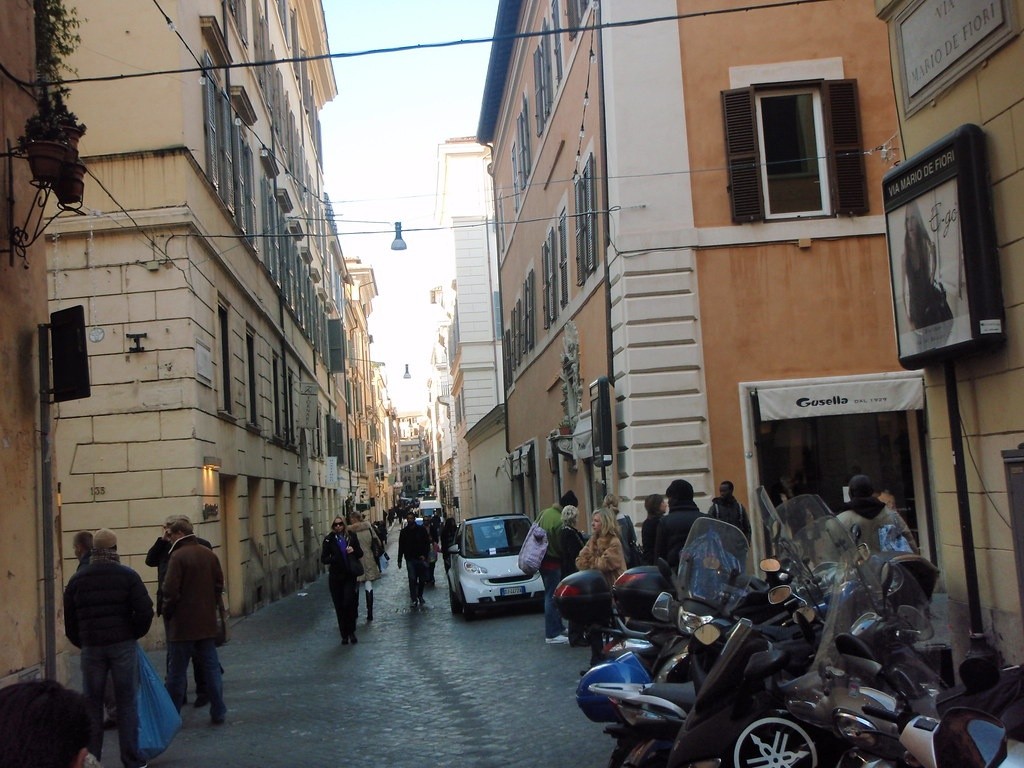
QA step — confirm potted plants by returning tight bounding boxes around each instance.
[20,94,88,204]
[557,421,574,435]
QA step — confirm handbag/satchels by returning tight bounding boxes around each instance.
[369,528,391,561]
[878,511,914,554]
[518,509,548,576]
[135,644,182,760]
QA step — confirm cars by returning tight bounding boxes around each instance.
[398,518,424,532]
[446,513,547,621]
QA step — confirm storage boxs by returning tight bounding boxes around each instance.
[554,569,615,628]
[612,564,674,623]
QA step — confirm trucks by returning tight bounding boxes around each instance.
[419,500,445,527]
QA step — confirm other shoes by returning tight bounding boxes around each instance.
[194,695,210,708]
[418,595,426,605]
[350,633,358,644]
[342,638,348,644]
[546,628,569,644]
[410,600,418,608]
[570,637,590,647]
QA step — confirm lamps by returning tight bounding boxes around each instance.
[205,456,222,468]
[391,222,406,249]
[404,364,410,378]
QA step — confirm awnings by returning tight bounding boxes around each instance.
[500,415,593,480]
[750,377,926,423]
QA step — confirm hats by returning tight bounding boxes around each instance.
[559,490,578,508]
[93,528,117,549]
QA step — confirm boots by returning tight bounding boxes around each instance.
[365,589,374,621]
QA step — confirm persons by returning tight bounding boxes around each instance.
[72,531,119,732]
[144,514,225,708]
[62,528,155,768]
[320,513,364,645]
[346,511,382,622]
[769,447,806,509]
[396,474,924,678]
[159,517,229,726]
[900,199,955,355]
[0,676,93,768]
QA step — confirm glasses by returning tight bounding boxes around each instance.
[334,522,344,527]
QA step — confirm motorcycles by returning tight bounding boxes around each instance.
[555,485,1023,768]
